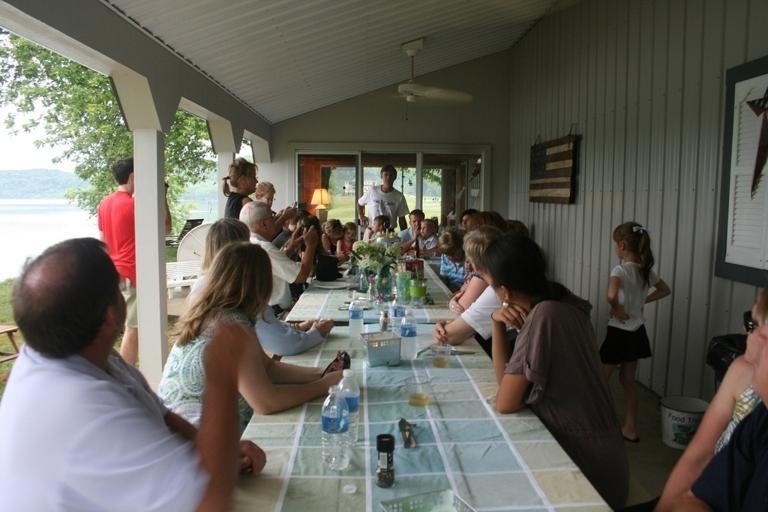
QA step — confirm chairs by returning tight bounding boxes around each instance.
[165,216,204,247]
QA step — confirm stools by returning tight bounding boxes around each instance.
[0,325,20,364]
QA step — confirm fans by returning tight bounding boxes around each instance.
[340,48,475,105]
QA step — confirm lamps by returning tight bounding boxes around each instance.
[310,188,332,221]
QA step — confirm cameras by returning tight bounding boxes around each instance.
[298,224,310,237]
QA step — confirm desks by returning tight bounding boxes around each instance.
[226,326,614,512]
[283,255,462,323]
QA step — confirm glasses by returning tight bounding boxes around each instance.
[743,308,759,334]
[319,351,352,377]
[260,196,277,202]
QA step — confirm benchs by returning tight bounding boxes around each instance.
[165,261,205,297]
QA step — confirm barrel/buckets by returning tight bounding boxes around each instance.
[658,396,711,451]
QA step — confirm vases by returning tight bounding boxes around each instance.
[373,268,397,307]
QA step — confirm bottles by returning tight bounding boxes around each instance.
[347,249,427,361]
[375,435,395,487]
[338,370,360,448]
[321,385,350,469]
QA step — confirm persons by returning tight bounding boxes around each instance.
[615,285,768,511]
[2,237,267,510]
[97,157,172,369]
[358,165,410,251]
[395,208,531,360]
[156,242,344,431]
[660,322,768,511]
[198,159,359,356]
[481,237,630,509]
[600,223,671,440]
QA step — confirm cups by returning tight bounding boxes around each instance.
[431,343,451,368]
[407,376,431,406]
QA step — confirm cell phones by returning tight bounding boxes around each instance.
[292,201,297,208]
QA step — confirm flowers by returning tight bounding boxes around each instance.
[346,224,414,276]
